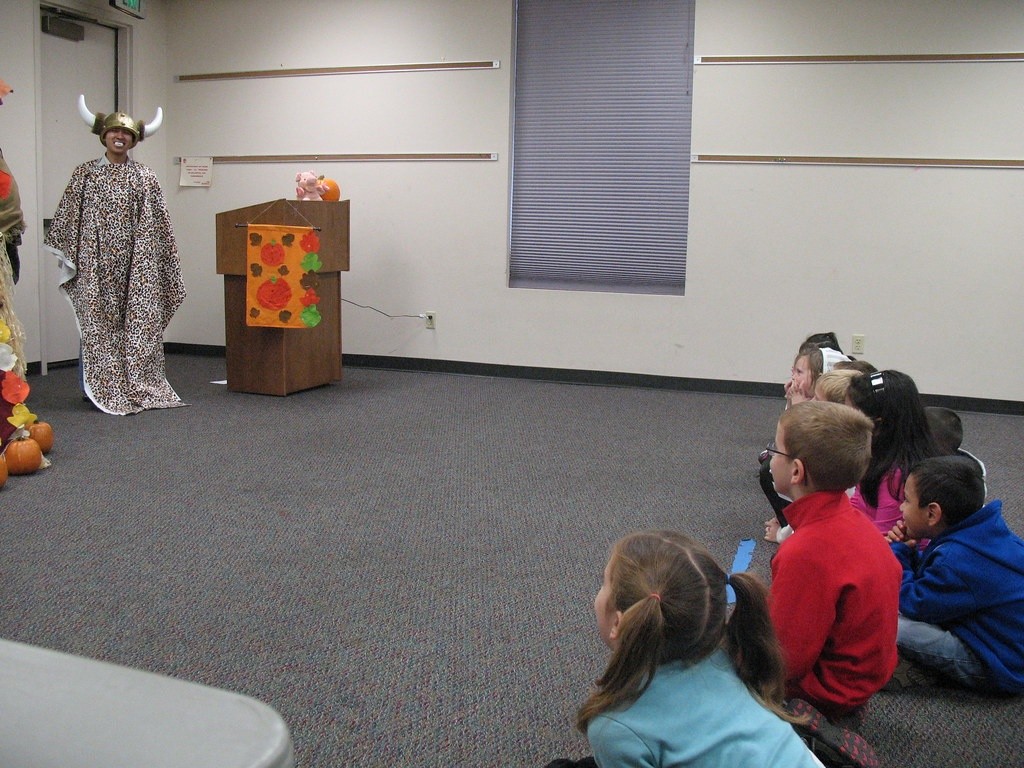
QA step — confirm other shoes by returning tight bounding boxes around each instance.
[784,698,878,768]
[881,649,938,697]
[758,450,769,464]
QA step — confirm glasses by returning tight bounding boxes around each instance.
[766,441,808,486]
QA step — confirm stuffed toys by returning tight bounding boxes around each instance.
[295,171,330,201]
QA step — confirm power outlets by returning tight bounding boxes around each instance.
[426,311,436,328]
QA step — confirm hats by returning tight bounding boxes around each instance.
[78,95,162,150]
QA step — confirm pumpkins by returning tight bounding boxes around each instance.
[26,419,54,454]
[0,455,9,488]
[4,436,41,475]
[315,174,340,201]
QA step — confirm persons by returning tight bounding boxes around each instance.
[0,78,26,285]
[729,401,903,768]
[759,332,965,552]
[544,532,824,768]
[879,456,1024,695]
[42,94,192,415]
